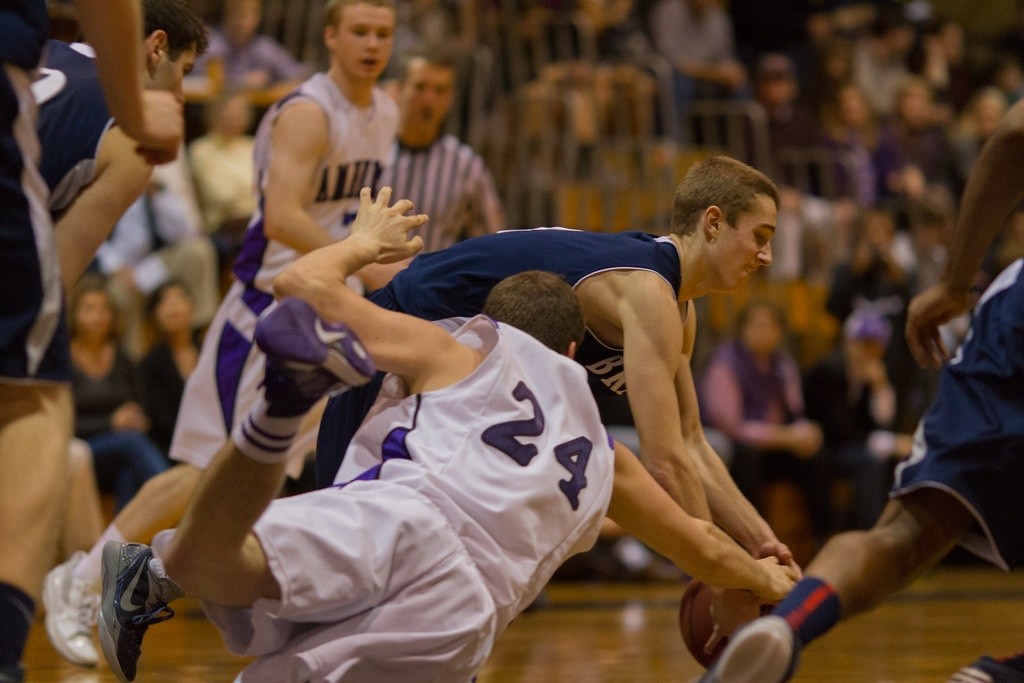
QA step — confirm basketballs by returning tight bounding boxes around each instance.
[679,575,776,671]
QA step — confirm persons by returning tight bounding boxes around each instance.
[383,36,510,263]
[161,185,801,683]
[695,97,1024,683]
[0,0,316,683]
[412,1,1023,532]
[39,0,409,666]
[95,153,806,683]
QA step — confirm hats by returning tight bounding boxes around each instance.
[841,304,892,343]
[754,52,799,79]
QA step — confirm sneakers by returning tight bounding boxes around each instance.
[42,550,98,666]
[251,297,375,417]
[698,617,799,682]
[948,654,1024,683]
[97,540,186,683]
